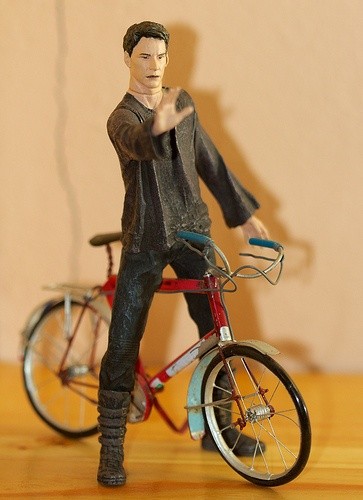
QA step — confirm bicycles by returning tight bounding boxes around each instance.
[17,231,312,488]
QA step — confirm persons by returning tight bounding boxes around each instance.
[98,21,270,486]
